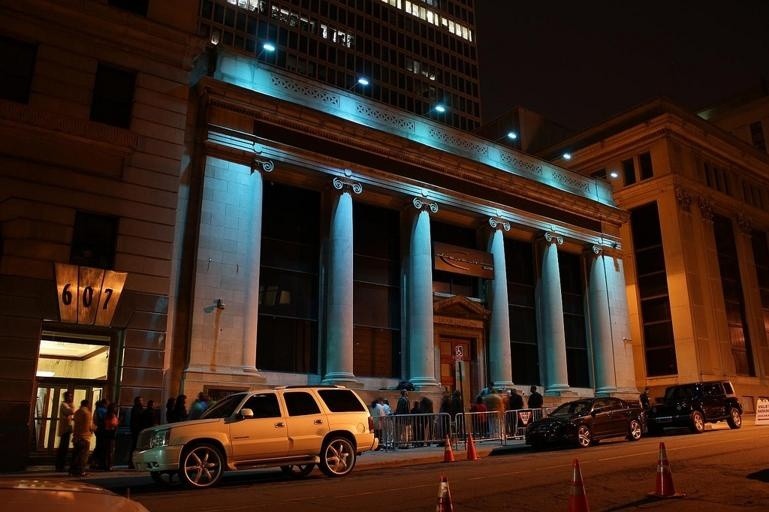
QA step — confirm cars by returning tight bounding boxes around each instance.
[0,479,148,512]
[525,397,648,451]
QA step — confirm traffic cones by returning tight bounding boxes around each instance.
[434,476,454,512]
[461,431,481,462]
[648,441,686,501]
[441,433,459,464]
[569,458,589,512]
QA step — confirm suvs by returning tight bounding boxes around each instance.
[646,380,744,435]
[132,385,379,487]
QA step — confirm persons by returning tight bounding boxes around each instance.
[639,387,651,409]
[371,381,543,451]
[54,390,217,478]
